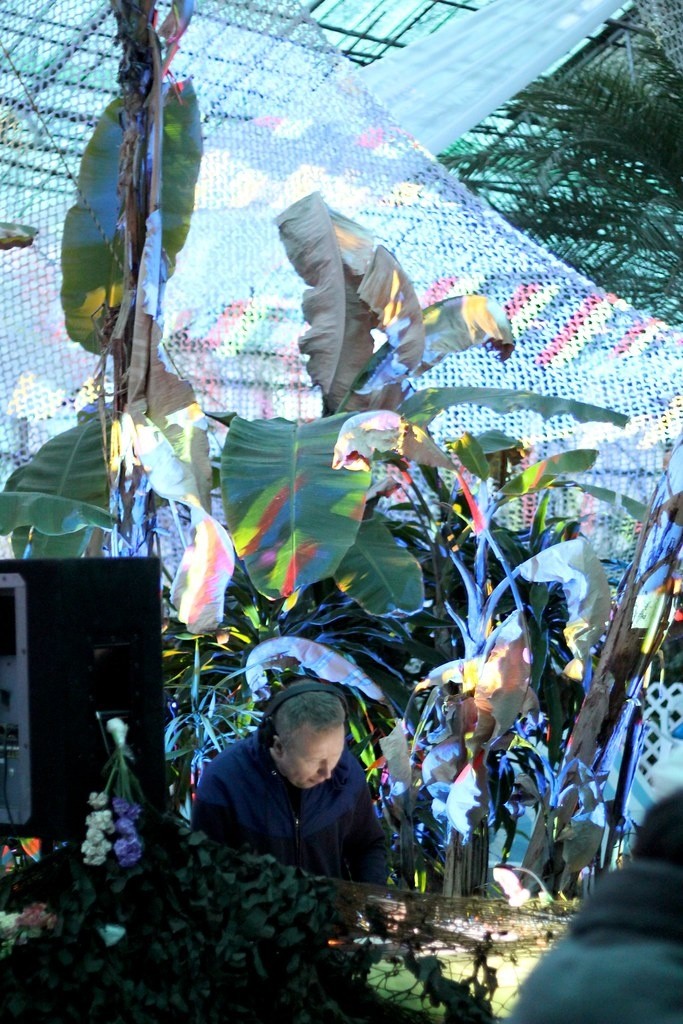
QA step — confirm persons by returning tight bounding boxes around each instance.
[188,677,392,886]
[501,786,682,1024]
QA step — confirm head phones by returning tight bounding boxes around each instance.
[258,683,350,749]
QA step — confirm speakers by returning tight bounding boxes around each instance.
[0,556,166,839]
